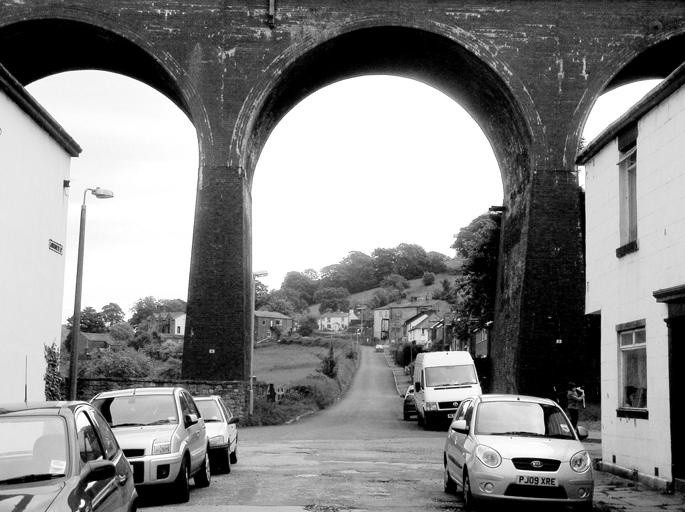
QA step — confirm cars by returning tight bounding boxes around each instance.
[375,344,384,352]
[440,392,597,509]
[0,399,141,512]
[187,392,241,473]
[400,384,418,421]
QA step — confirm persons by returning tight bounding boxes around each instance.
[567,382,583,430]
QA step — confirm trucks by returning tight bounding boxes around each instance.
[411,348,483,427]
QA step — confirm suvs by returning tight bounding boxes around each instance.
[87,385,214,501]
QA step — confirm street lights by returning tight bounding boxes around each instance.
[68,187,114,397]
[356,328,359,347]
[248,269,269,415]
